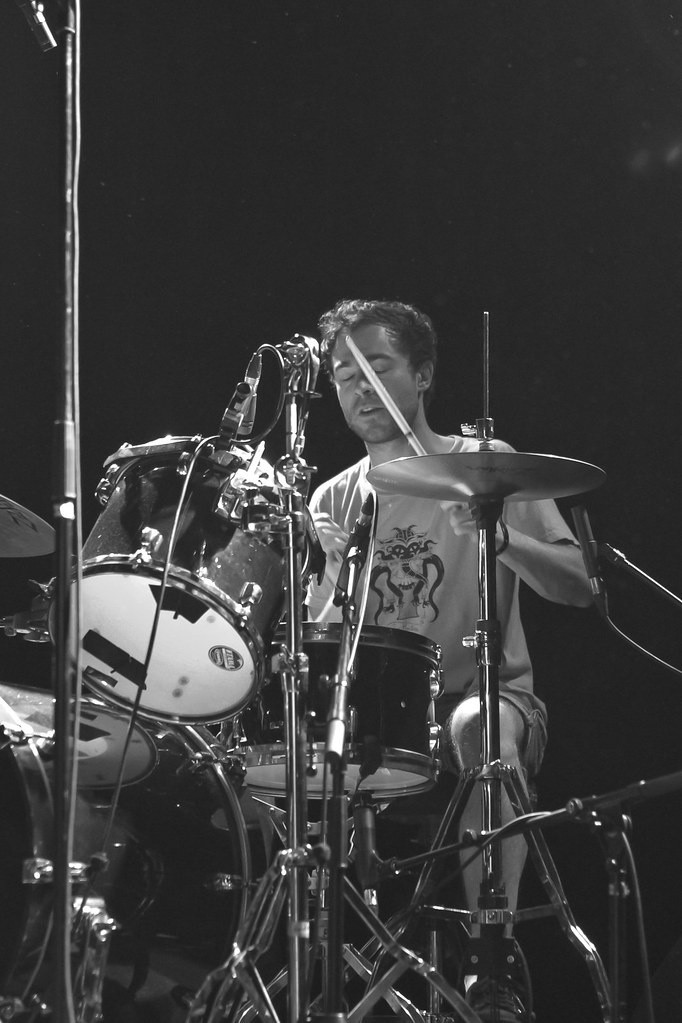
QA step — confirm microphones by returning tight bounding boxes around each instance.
[222,352,263,436]
[16,0,57,52]
[351,791,379,917]
[571,505,609,618]
[334,490,377,608]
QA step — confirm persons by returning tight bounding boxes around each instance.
[304,302,601,1022]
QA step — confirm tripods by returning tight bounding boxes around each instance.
[188,333,623,1023]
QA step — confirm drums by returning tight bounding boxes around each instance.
[48,433,328,726]
[0,681,256,1023]
[215,621,447,802]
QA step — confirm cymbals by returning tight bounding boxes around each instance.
[0,493,57,558]
[365,450,608,505]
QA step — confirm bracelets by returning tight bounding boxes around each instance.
[496,519,509,556]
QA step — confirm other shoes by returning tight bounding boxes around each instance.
[463,973,523,1023]
